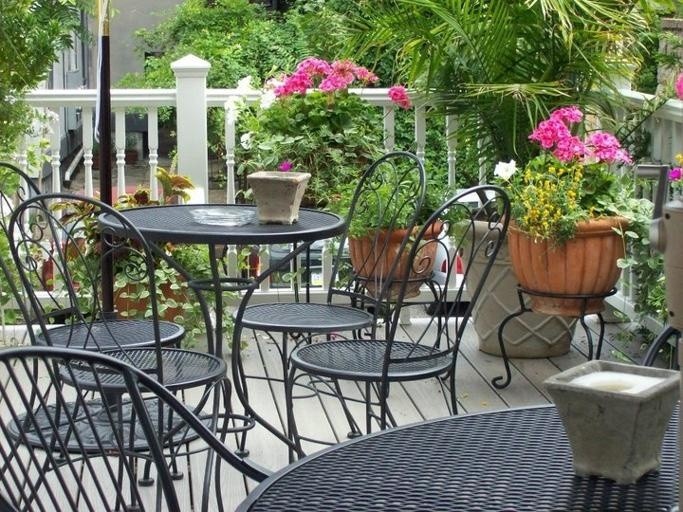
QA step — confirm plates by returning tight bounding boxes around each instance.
[189,208,256,227]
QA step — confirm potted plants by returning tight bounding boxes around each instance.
[316,135,470,301]
[328,0,683,359]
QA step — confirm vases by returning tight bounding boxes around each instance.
[664,200,682,332]
[497,213,632,317]
[543,359,681,486]
[290,143,368,210]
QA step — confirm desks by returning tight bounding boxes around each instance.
[97,202,348,512]
[234,399,682,512]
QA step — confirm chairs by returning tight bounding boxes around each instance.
[6,191,227,512]
[231,151,428,457]
[288,182,511,463]
[1,346,273,512]
[0,162,186,488]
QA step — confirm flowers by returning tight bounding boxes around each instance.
[488,104,670,363]
[667,73,682,199]
[223,55,413,204]
